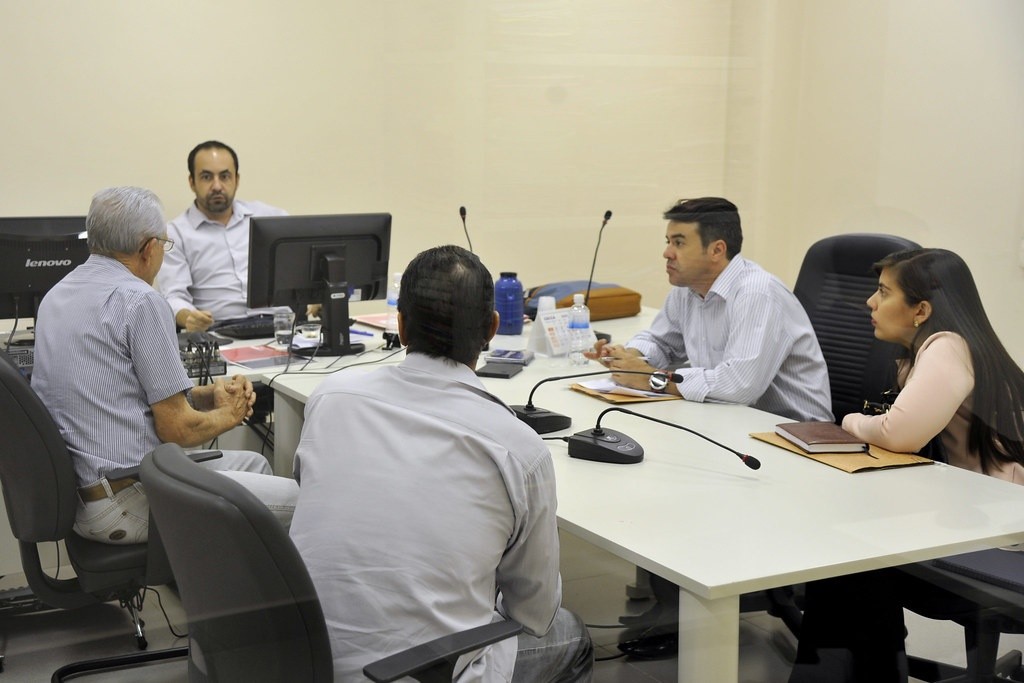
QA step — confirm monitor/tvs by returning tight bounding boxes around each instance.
[246,213,394,357]
[0,216,89,344]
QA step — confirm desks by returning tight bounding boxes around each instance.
[0,283,1024,683]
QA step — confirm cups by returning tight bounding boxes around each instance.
[273,311,295,345]
[301,324,321,347]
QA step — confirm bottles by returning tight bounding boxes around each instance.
[566,294,590,367]
[494,272,523,335]
[385,273,402,333]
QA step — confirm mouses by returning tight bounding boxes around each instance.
[188,331,212,343]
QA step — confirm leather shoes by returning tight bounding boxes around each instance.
[618,631,679,660]
[619,605,654,625]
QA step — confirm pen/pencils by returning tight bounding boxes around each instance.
[598,357,652,360]
[349,330,373,336]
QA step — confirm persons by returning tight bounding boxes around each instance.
[789,246,1024,683]
[579,197,836,661]
[288,245,596,683]
[28,185,298,536]
[154,141,324,334]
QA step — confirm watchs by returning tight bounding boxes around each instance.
[648,368,669,392]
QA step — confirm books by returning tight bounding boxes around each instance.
[776,420,880,460]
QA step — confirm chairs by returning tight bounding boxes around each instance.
[739,233,925,640]
[0,355,223,683]
[902,547,1024,683]
[139,443,523,683]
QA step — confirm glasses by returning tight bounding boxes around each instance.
[139,237,175,253]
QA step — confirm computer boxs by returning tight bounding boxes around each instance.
[0,333,36,383]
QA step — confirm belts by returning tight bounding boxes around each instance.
[78,473,141,503]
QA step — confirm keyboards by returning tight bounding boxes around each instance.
[216,318,357,339]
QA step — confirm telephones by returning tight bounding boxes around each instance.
[216,313,275,339]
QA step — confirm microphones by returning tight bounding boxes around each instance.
[542,437,569,443]
[584,209,612,306]
[510,370,684,434]
[568,407,762,471]
[459,207,472,252]
